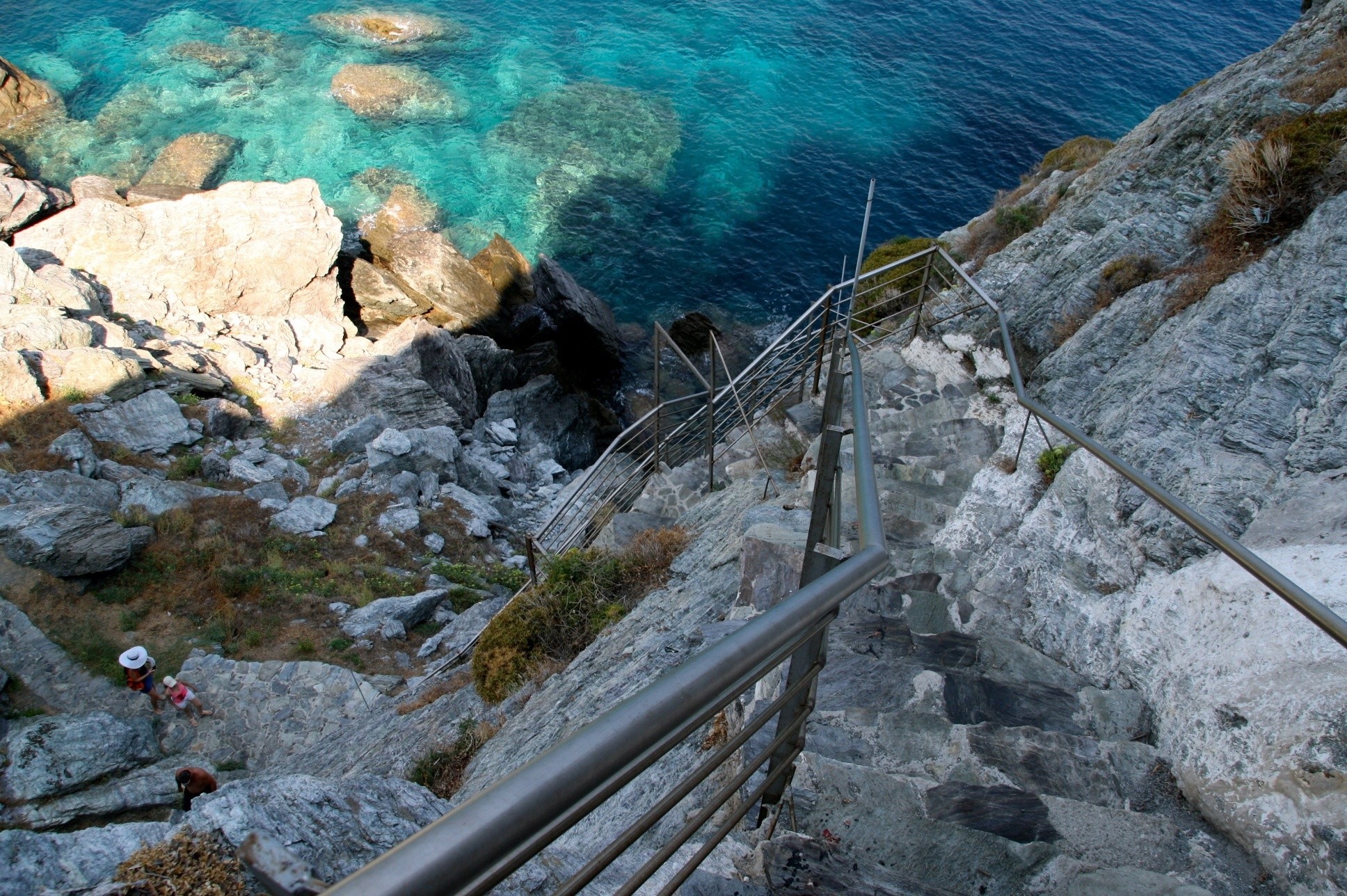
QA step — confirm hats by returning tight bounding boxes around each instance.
[163,676,177,688]
[179,770,190,783]
[119,646,148,668]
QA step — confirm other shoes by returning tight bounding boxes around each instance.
[189,717,198,727]
[161,695,166,700]
[201,710,213,715]
[155,710,166,714]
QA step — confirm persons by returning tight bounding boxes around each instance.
[163,675,213,726]
[119,646,166,715]
[175,767,217,812]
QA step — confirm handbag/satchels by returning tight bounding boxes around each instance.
[127,671,144,691]
